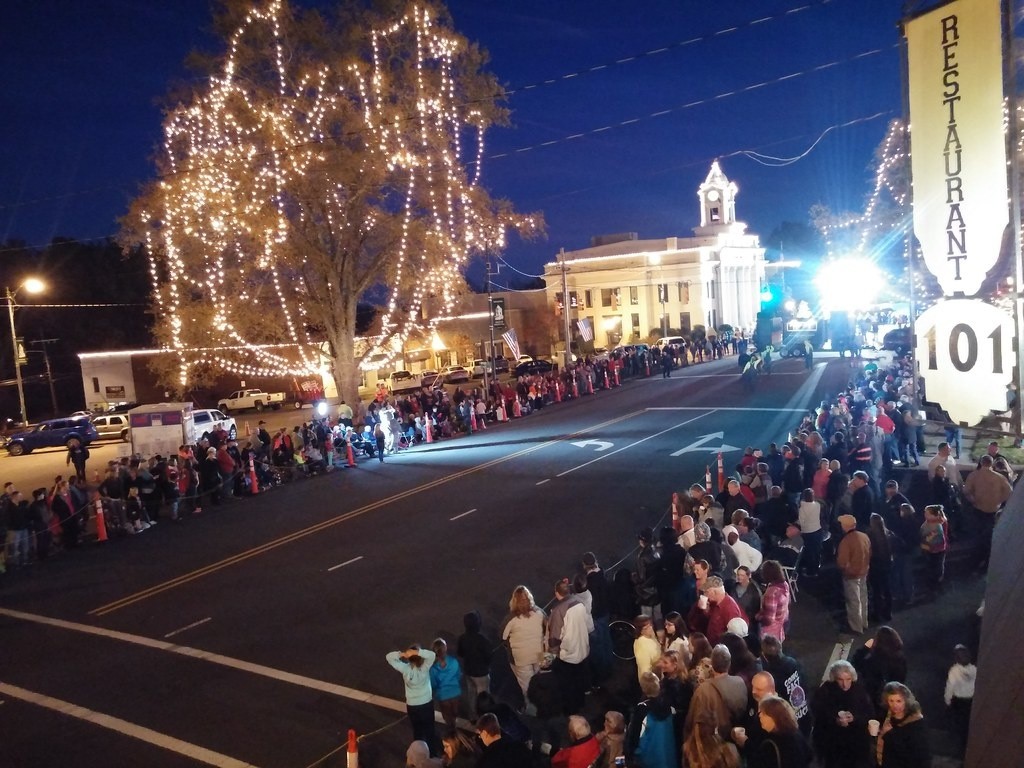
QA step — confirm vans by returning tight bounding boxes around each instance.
[609,343,650,361]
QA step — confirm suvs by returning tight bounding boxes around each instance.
[93,415,131,443]
[880,328,918,355]
[3,416,98,456]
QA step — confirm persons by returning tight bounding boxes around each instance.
[943,644,978,742]
[804,341,813,369]
[430,638,462,736]
[0,335,747,595]
[836,515,871,634]
[754,559,789,643]
[660,352,671,378]
[964,455,1013,567]
[387,307,1015,768]
[67,439,89,483]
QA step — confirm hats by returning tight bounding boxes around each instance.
[637,527,656,543]
[786,521,802,532]
[712,644,731,664]
[838,514,857,526]
[699,575,724,591]
[582,551,596,566]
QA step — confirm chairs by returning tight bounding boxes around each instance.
[350,427,419,462]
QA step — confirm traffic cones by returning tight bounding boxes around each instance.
[244,421,251,436]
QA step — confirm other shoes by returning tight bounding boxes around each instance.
[879,606,893,620]
[870,607,881,622]
[840,621,868,635]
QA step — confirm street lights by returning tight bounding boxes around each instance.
[6,279,45,431]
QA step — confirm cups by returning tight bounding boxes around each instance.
[699,594,708,610]
[867,719,880,737]
[838,710,849,728]
[700,505,705,511]
[734,727,746,739]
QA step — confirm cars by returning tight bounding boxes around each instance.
[651,337,688,357]
[461,355,532,379]
[544,351,577,364]
[585,347,611,365]
[440,365,470,384]
[508,360,557,381]
[70,403,140,417]
[420,369,443,390]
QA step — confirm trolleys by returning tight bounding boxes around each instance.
[288,390,327,409]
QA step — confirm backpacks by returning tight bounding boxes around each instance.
[648,557,679,593]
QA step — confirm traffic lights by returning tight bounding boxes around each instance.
[761,291,772,304]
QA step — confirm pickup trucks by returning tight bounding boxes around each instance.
[192,409,237,442]
[218,388,286,414]
[384,370,421,396]
